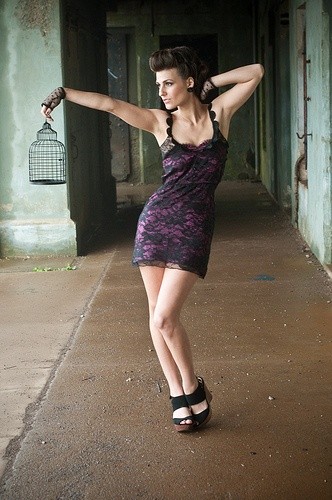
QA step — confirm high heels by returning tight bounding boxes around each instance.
[169,394,193,431]
[183,376,213,428]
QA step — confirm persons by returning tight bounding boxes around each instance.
[40,45,266,432]
